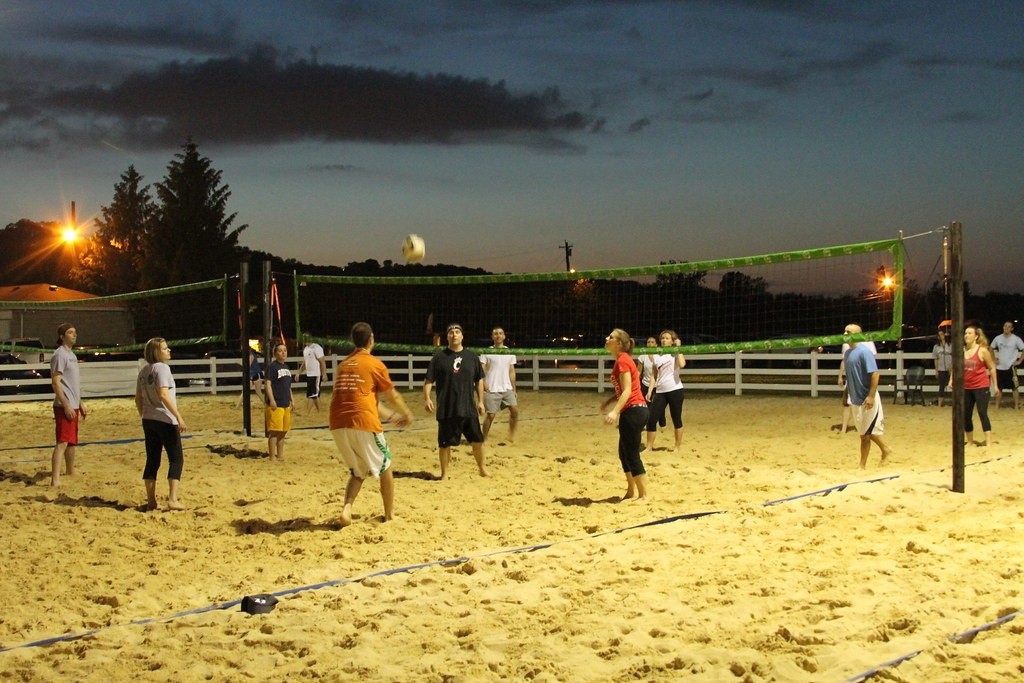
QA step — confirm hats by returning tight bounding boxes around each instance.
[56,322,75,345]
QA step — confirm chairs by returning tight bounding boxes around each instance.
[893,366,926,406]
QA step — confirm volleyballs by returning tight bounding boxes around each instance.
[403,233,426,261]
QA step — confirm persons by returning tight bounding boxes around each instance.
[49,323,86,486]
[480,328,518,445]
[637,336,666,432]
[329,322,413,526]
[236,348,265,408]
[600,327,649,501]
[991,322,1024,409]
[295,331,327,415]
[643,330,686,454]
[839,342,877,433]
[423,323,492,480]
[933,330,952,406]
[844,323,891,470]
[947,326,1000,448]
[265,343,294,462]
[136,337,187,511]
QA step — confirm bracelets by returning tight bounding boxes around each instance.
[387,412,396,422]
